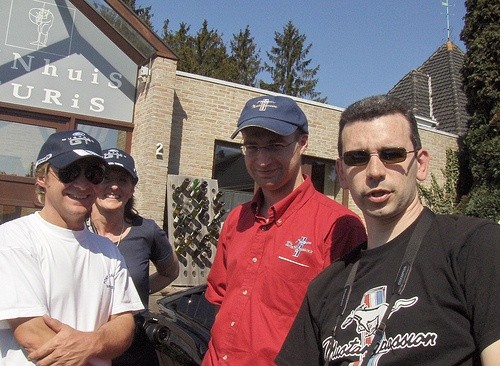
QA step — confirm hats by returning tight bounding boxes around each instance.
[231,96,308,138]
[35,130,109,171]
[99,148,137,179]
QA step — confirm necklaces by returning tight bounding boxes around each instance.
[115,216,125,247]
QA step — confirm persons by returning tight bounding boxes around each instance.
[202,94,367,366]
[84,148,180,314]
[276,95,500,366]
[0,130,145,366]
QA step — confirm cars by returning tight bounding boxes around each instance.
[143,283,221,366]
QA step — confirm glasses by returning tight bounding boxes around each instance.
[339,148,419,165]
[239,139,298,156]
[46,165,105,185]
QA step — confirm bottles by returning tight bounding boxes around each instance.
[173,177,226,259]
